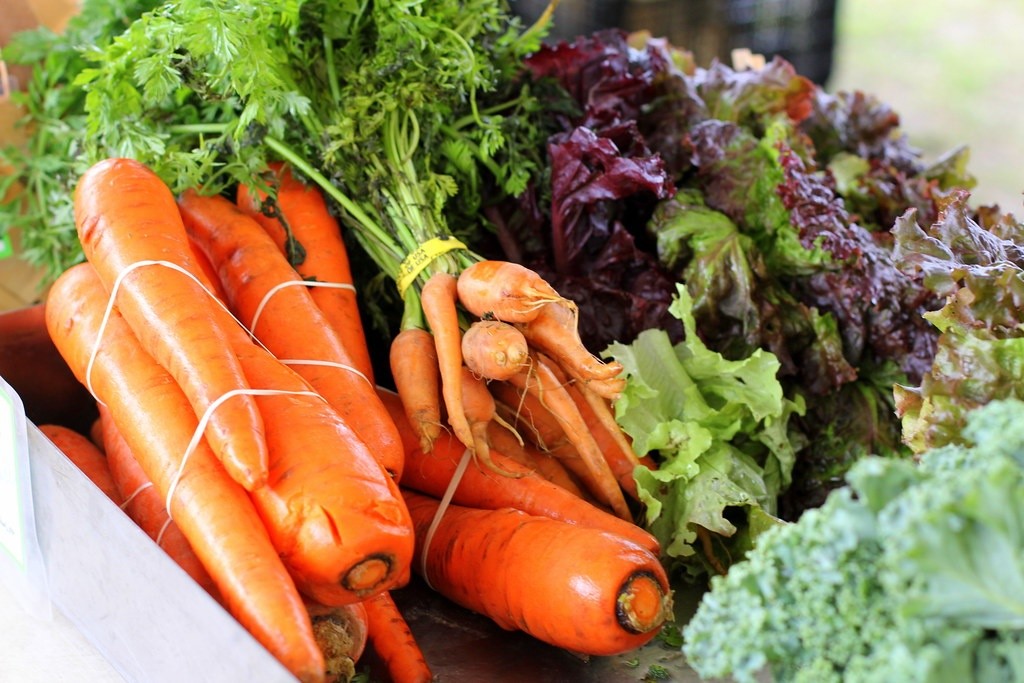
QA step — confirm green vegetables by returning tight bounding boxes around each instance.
[469,20,1024,683]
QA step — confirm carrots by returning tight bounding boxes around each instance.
[30,156,674,683]
